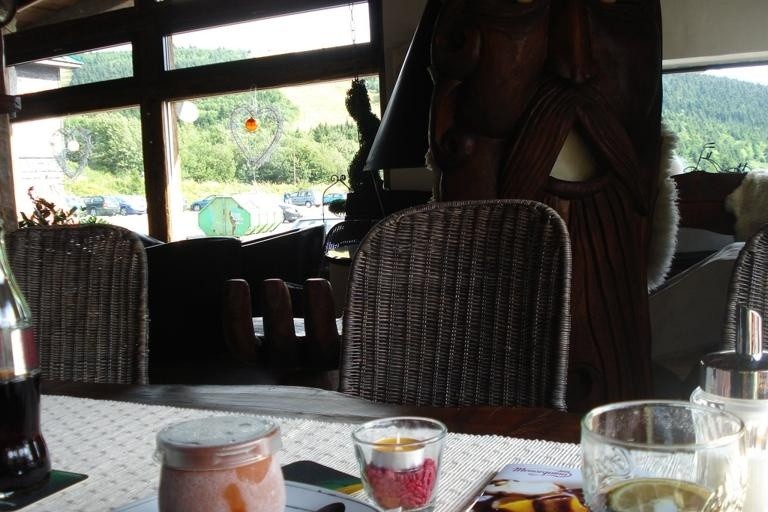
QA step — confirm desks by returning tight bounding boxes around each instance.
[1,384,764,511]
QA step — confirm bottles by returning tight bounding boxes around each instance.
[1,219,52,492]
[687,304,767,512]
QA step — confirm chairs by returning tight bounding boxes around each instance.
[3,222,152,386]
[136,224,324,365]
[337,197,573,410]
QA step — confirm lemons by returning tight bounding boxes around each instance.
[606,479,719,510]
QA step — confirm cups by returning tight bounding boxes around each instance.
[352,413,447,512]
[578,401,745,512]
